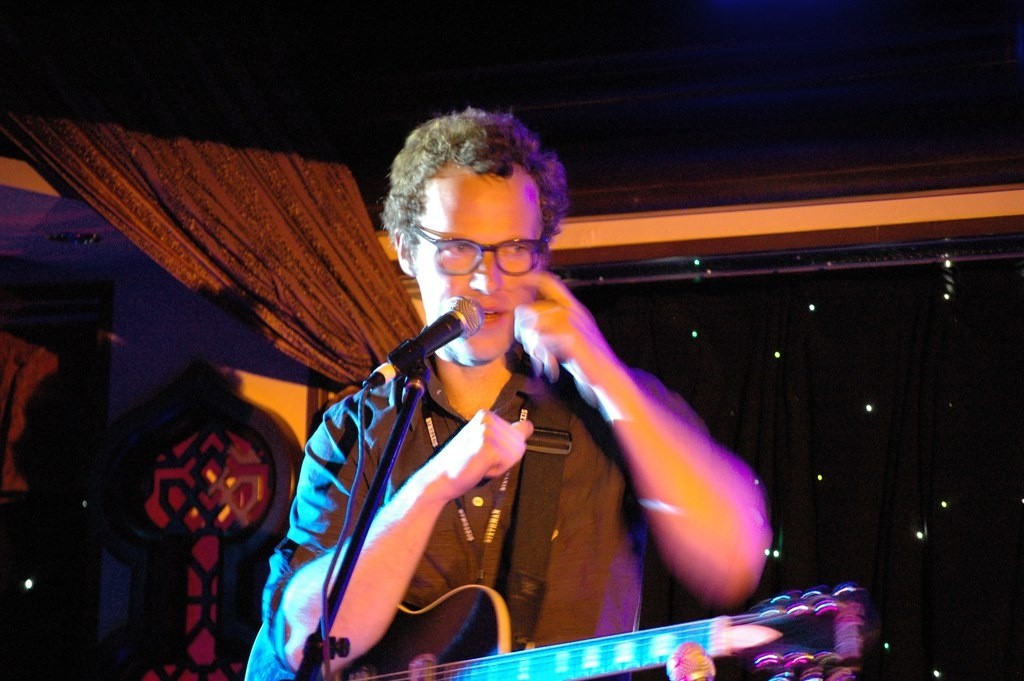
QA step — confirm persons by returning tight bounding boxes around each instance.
[263,107,774,681]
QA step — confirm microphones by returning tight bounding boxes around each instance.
[363,295,485,392]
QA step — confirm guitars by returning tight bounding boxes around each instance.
[243,584,881,681]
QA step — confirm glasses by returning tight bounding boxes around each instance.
[408,223,549,276]
